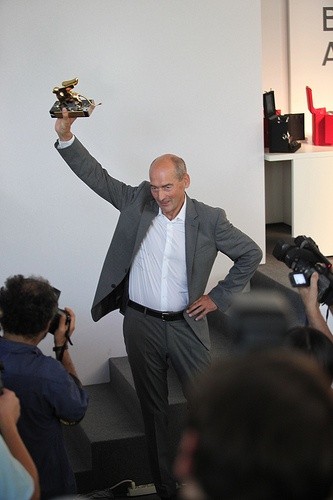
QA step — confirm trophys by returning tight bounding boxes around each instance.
[49,77,102,118]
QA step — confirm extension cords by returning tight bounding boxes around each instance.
[128,483,156,496]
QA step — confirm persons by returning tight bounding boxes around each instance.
[54,107,263,500]
[170,271,333,500]
[0,274,88,500]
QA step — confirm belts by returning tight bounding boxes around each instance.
[126,299,185,322]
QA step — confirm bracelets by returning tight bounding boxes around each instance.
[52,344,69,352]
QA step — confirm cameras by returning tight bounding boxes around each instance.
[48,309,71,335]
